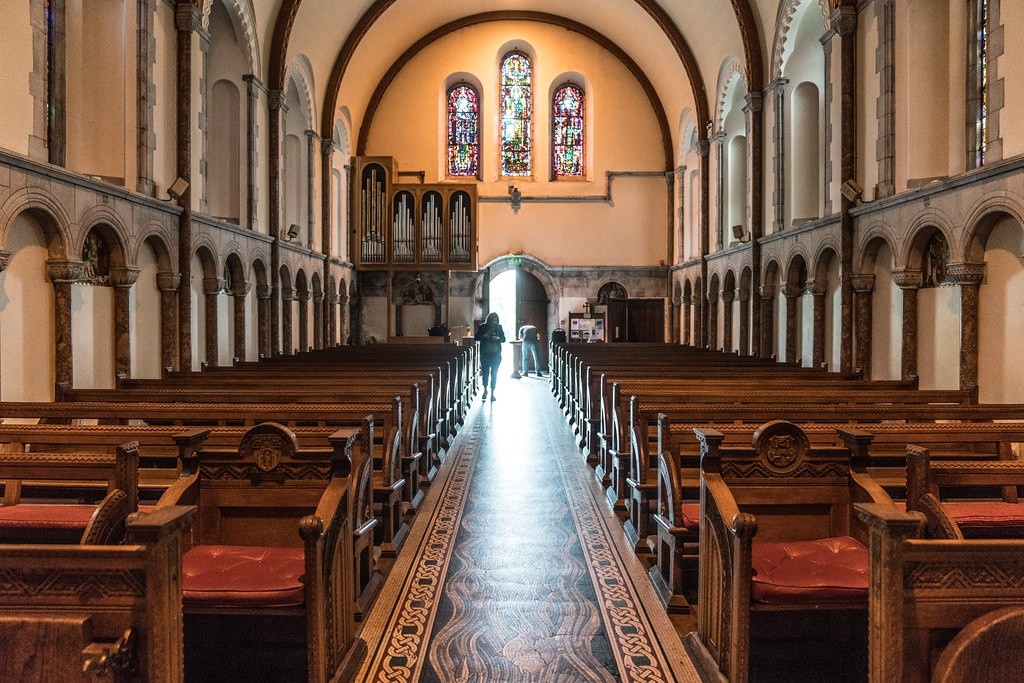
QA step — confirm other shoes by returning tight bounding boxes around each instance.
[490,395,497,401]
[522,372,529,377]
[481,390,489,399]
[536,370,543,377]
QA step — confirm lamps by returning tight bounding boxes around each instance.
[153,177,189,201]
[840,179,878,203]
[732,225,750,243]
[280,224,300,242]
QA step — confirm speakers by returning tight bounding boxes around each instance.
[287,225,301,238]
[167,177,189,200]
[733,225,744,238]
[840,179,863,202]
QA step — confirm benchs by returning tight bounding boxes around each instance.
[0,337,480,683]
[550,341,1024,683]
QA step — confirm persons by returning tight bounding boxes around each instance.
[474,312,505,401]
[518,325,541,377]
